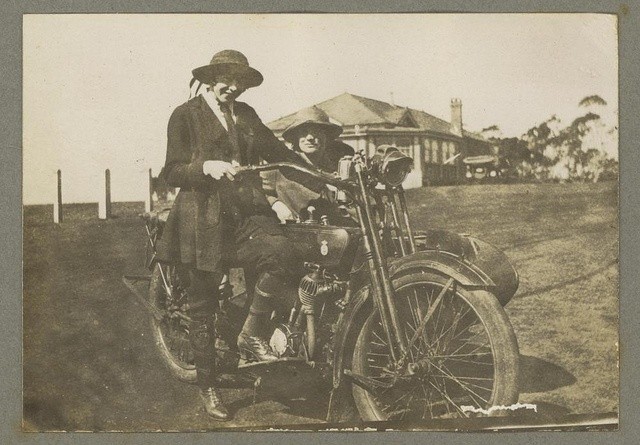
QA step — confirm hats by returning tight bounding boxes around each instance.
[282,106,343,142]
[191,50,263,87]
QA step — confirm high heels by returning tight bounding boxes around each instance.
[237,330,278,364]
[199,384,231,422]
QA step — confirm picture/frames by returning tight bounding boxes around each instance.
[0,0,640,445]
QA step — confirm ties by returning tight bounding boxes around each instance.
[218,102,243,162]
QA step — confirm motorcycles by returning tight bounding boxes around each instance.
[121,144,523,424]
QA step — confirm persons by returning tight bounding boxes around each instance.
[153,49,340,423]
[259,104,354,226]
